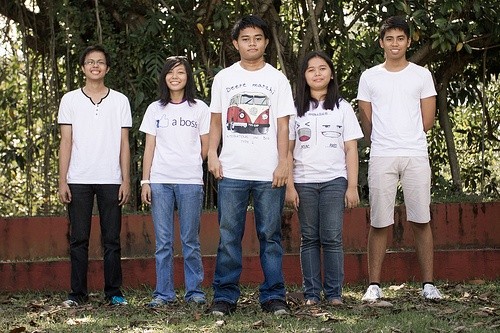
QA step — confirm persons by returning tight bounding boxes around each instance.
[138,56,210,309]
[285,52,365,307]
[57,45,133,308]
[357,16,445,300]
[207,16,296,316]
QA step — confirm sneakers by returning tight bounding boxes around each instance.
[361,285,380,302]
[423,283,443,299]
[205,301,237,316]
[260,299,292,315]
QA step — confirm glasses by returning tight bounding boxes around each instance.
[84,60,106,65]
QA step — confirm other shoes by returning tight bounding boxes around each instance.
[112,296,128,306]
[328,298,343,305]
[148,297,166,307]
[306,296,319,305]
[64,300,79,307]
[192,296,206,304]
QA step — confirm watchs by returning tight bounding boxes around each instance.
[140,180,150,186]
